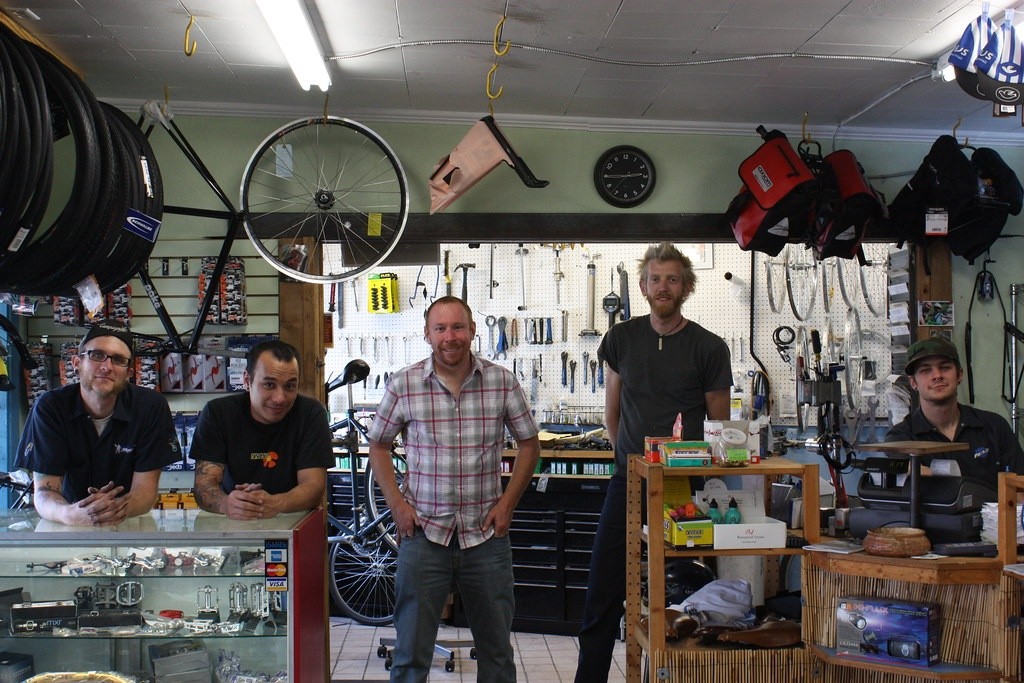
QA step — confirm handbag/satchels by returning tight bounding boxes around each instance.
[950,124,1024,268]
[811,151,879,260]
[888,134,978,249]
[729,126,818,258]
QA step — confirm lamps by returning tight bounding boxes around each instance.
[931,2,1024,84]
[254,0,333,92]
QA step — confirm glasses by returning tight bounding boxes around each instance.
[80,348,131,367]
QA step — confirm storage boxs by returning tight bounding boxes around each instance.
[837,596,943,668]
[644,420,761,468]
[714,509,787,550]
[0,587,214,683]
[663,501,714,546]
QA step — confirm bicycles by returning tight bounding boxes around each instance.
[82,97,410,358]
[320,360,408,625]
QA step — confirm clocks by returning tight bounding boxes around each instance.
[593,144,658,207]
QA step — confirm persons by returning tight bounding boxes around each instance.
[188,342,335,520]
[884,336,1024,492]
[369,295,541,683]
[12,319,182,527]
[573,240,734,683]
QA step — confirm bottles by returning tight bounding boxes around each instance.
[699,496,740,524]
[714,428,748,467]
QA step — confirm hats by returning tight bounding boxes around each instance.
[974,22,1024,106]
[79,318,133,355]
[947,16,998,101]
[905,336,960,376]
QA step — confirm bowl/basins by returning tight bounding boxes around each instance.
[863,526,931,556]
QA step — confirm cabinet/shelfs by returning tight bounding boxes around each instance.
[811,551,1003,680]
[890,242,952,373]
[626,453,821,683]
[0,507,330,683]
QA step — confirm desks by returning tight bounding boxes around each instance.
[856,441,970,529]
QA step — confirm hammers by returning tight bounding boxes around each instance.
[580,264,598,336]
[455,263,476,304]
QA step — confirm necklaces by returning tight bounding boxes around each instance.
[649,315,683,350]
